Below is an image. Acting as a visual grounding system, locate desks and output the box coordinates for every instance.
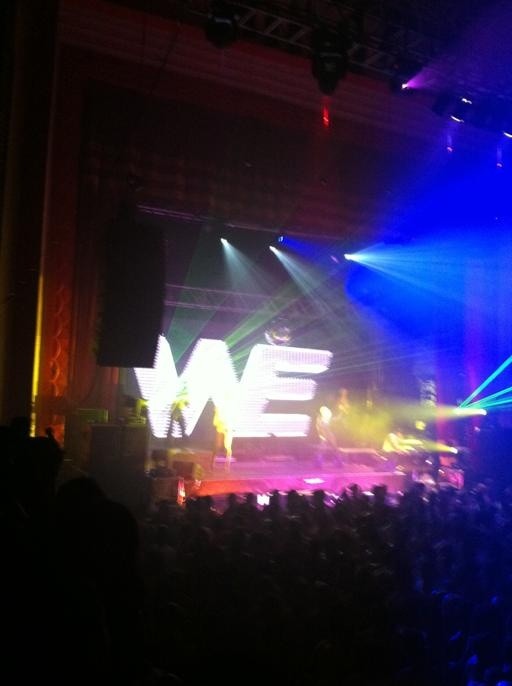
[63,417,108,459]
[89,423,148,471]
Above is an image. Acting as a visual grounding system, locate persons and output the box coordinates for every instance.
[211,396,234,474]
[337,387,354,417]
[0,414,512,686]
[166,386,190,438]
[315,405,346,470]
